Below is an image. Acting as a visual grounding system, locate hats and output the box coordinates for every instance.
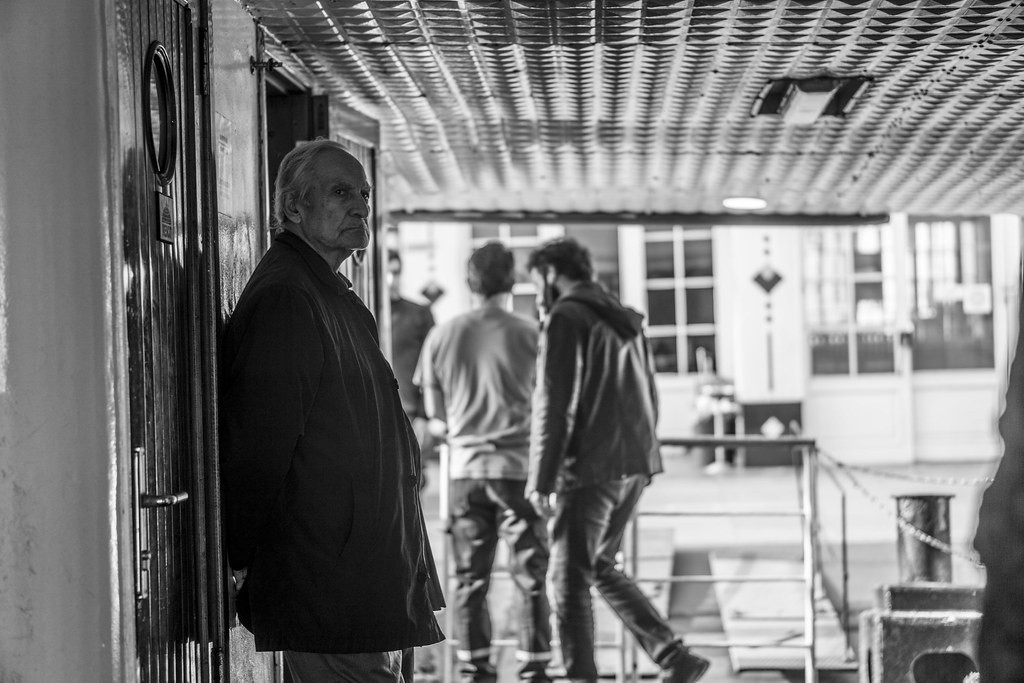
[468,243,514,294]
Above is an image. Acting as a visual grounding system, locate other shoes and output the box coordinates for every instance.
[659,642,707,683]
[516,661,552,683]
[457,662,497,683]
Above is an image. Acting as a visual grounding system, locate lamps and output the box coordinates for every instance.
[751,79,869,125]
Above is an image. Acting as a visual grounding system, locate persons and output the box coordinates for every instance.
[410,241,552,683]
[218,135,449,683]
[385,247,437,490]
[525,234,709,683]
